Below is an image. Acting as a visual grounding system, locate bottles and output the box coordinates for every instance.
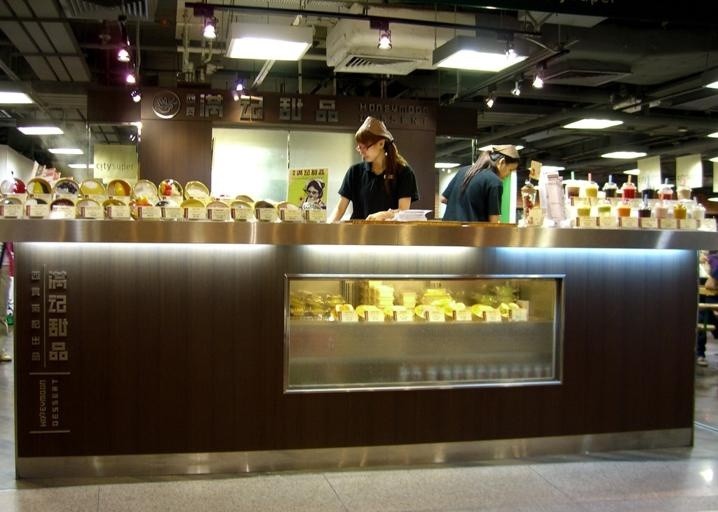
[546,171,566,223]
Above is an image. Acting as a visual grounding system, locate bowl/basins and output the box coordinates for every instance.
[27,177,53,220]
[207,195,327,223]
[157,179,185,220]
[184,181,210,219]
[106,178,131,220]
[52,179,80,221]
[131,180,162,220]
[79,179,106,220]
[0,176,26,218]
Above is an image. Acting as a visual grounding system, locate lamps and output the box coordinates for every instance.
[432,26,531,74]
[224,7,316,62]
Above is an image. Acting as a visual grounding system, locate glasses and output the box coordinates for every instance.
[355,140,380,152]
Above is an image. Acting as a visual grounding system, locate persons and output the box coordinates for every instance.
[332,115,421,222]
[298,177,328,209]
[439,142,521,224]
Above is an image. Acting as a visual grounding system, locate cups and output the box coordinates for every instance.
[563,171,707,233]
[520,184,537,220]
